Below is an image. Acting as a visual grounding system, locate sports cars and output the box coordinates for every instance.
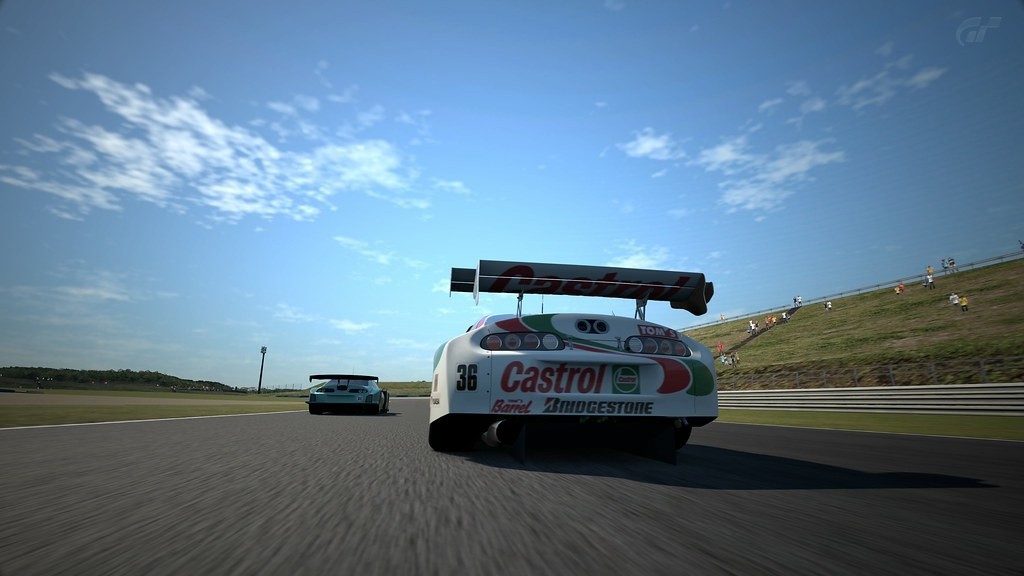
[428,259,720,458]
[305,374,390,416]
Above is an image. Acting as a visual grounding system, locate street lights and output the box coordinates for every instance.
[258,345,268,394]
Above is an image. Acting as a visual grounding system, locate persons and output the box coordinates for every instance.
[721,313,725,323]
[747,310,790,336]
[793,296,802,308]
[88,379,95,385]
[823,300,832,312]
[170,384,222,392]
[949,292,969,312]
[1020,242,1024,250]
[19,385,22,390]
[717,341,740,368]
[922,256,960,290]
[157,384,159,387]
[893,281,905,295]
[105,380,112,385]
[31,375,54,389]
